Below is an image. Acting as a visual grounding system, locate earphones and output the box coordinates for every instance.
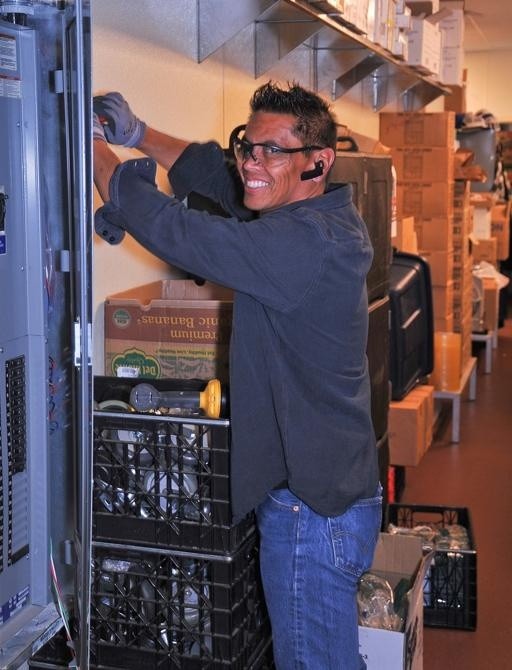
[300,161,325,180]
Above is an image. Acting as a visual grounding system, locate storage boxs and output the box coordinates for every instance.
[303,1,465,89]
[103,276,236,384]
[357,530,437,670]
[329,112,512,469]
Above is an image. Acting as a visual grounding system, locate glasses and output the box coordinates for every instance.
[227,124,325,166]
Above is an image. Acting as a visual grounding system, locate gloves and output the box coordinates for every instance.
[90,91,146,146]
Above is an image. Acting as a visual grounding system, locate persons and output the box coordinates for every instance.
[93,81,385,670]
